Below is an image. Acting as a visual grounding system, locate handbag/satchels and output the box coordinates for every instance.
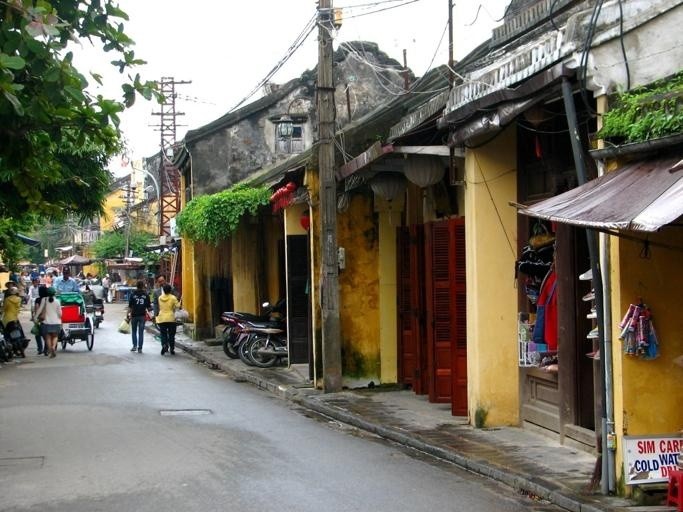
[118,317,132,335]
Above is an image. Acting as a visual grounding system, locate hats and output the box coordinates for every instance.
[5,280,18,288]
[9,287,19,295]
[63,267,71,274]
[47,287,56,295]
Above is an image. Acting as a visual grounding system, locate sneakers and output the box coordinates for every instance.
[161,345,175,355]
[130,346,143,354]
[38,348,56,358]
[578,267,600,362]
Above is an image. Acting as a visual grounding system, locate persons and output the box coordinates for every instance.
[137,273,146,285]
[153,274,175,352]
[147,274,154,288]
[126,281,153,354]
[156,284,182,355]
[1,265,111,359]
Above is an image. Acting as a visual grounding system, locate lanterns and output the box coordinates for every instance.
[403,154,445,217]
[271,181,297,212]
[371,174,408,225]
[300,215,310,232]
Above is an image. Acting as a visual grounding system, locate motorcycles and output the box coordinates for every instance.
[94,299,105,328]
[0,321,31,362]
[222,302,287,368]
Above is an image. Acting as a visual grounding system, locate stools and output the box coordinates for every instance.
[667,470,682,512]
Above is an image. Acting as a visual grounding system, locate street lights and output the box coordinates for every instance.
[131,166,160,237]
[272,97,342,392]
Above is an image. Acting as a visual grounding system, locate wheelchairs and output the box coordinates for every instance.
[59,292,94,350]
